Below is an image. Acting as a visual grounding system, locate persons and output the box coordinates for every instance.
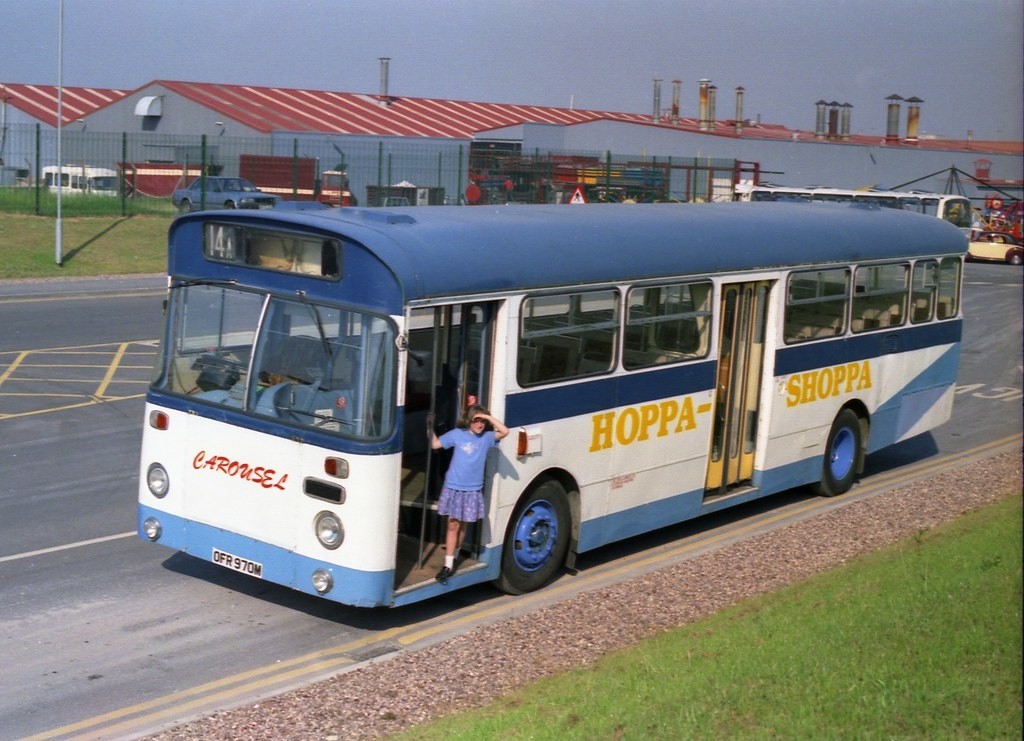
[426,404,509,580]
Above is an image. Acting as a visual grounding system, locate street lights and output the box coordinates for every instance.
[0,96,13,163]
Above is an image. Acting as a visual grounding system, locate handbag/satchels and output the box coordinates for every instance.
[195,367,240,391]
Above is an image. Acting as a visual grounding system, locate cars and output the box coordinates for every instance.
[963,231,1024,265]
[172,176,281,213]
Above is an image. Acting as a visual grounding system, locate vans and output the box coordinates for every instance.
[41,165,119,198]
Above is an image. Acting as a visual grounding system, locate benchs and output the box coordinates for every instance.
[445,281,953,383]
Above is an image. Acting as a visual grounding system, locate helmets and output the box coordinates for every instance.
[506,175,511,179]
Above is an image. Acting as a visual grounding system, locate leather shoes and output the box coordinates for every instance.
[435,557,460,580]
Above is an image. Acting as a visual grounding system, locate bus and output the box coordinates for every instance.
[135,202,972,609]
[735,184,974,247]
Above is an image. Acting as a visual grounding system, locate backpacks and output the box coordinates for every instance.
[549,347,570,379]
[274,378,354,437]
